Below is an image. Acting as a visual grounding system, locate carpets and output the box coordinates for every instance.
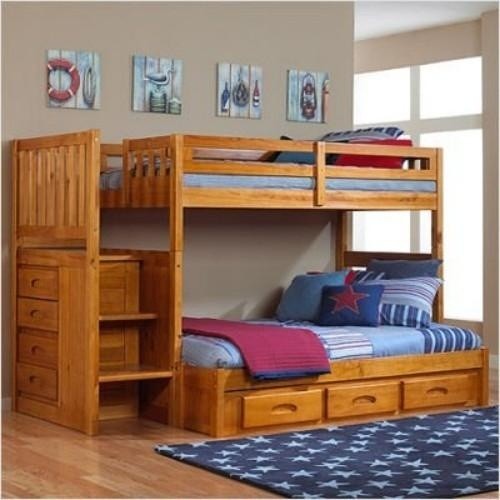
[155,404,499,498]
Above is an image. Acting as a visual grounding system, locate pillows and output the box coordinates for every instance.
[259,123,411,170]
[275,257,444,330]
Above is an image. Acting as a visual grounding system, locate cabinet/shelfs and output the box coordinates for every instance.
[14,256,141,422]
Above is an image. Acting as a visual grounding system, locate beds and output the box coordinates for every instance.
[100,131,491,438]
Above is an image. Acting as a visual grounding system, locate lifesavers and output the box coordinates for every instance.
[46,59,80,100]
[82,65,97,106]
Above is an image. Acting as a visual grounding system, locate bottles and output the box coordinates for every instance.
[220,79,232,113]
[252,79,260,109]
